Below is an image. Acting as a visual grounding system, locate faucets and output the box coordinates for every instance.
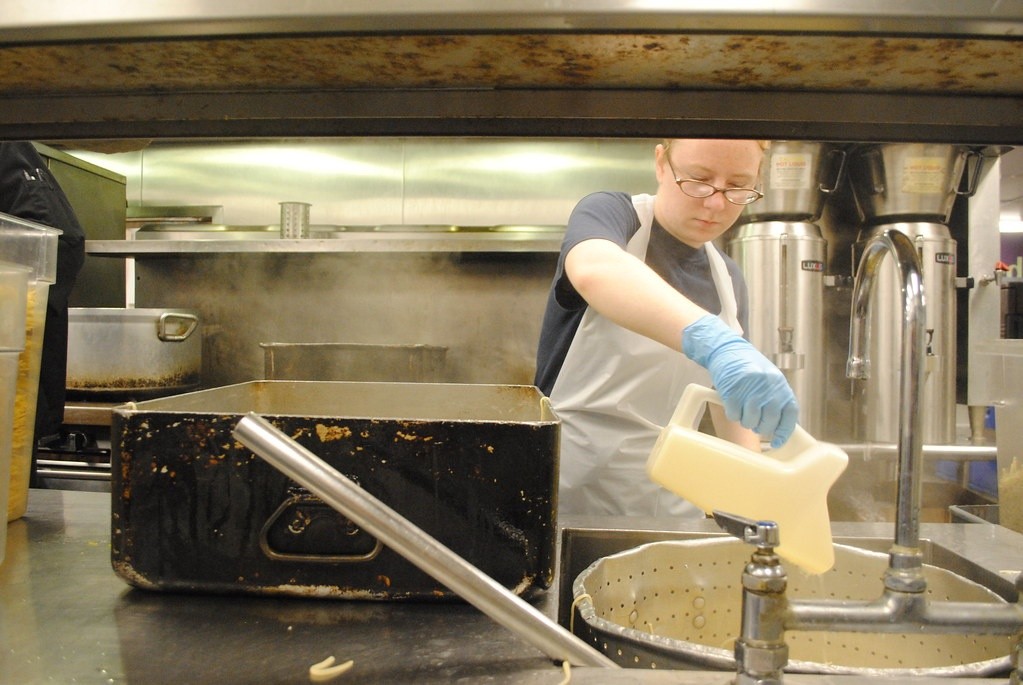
[844,225,935,636]
[981,262,1011,290]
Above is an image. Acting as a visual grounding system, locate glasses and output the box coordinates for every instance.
[664,146,765,206]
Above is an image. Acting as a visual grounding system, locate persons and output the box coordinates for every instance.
[532,135,798,536]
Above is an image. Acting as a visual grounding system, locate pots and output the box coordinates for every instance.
[259,339,452,385]
[67,306,202,393]
[109,377,565,603]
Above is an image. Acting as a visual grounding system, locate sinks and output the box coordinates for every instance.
[551,515,1023,685]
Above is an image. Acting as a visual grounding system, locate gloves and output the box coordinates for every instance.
[682,314,798,449]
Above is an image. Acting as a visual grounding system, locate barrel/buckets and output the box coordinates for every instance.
[718,218,830,443]
[844,221,958,449]
